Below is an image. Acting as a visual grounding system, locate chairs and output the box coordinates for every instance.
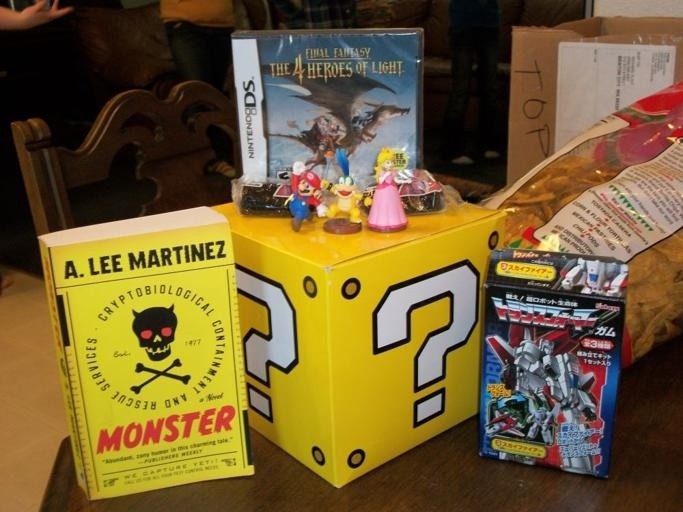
[10,79,243,238]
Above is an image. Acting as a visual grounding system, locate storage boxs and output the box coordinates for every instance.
[203,203,509,489]
[507,15,683,186]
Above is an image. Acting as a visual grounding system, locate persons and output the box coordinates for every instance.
[285,162,328,232]
[366,147,407,231]
[319,176,371,234]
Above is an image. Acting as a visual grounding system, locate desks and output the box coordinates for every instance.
[39,329,683,512]
